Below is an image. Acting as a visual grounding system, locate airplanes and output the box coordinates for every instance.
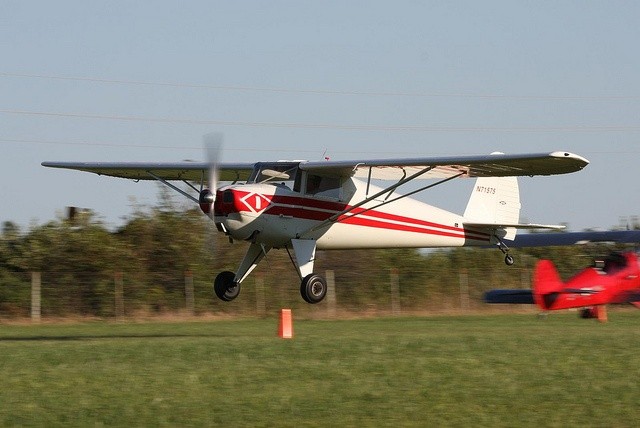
[41,151,590,306]
[481,229,640,323]
